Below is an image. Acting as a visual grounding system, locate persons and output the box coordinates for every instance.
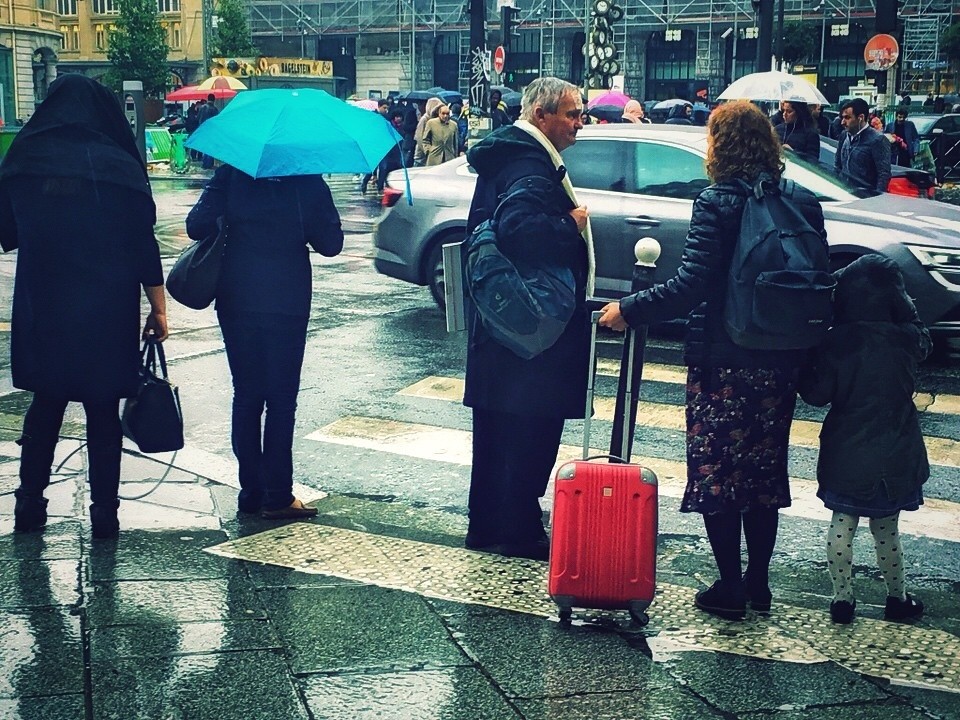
[463,76,597,562]
[801,253,933,623]
[580,92,936,166]
[884,109,919,167]
[361,94,467,193]
[835,98,891,192]
[0,74,169,541]
[491,90,513,132]
[600,100,831,618]
[186,163,344,519]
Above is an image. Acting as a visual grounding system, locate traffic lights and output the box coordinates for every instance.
[876,0,906,43]
[500,6,521,44]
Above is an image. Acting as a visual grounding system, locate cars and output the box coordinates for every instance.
[904,114,960,165]
[373,124,960,338]
[818,135,936,203]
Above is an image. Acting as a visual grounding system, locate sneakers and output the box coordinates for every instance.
[741,572,773,611]
[695,572,746,617]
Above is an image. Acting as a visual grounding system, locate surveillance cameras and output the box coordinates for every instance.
[721,28,733,38]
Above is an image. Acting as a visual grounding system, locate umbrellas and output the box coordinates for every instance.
[406,90,447,104]
[426,87,446,93]
[716,71,831,107]
[490,85,523,106]
[165,76,414,205]
[586,92,632,108]
[693,104,712,113]
[436,91,462,97]
[653,99,694,109]
[587,105,624,123]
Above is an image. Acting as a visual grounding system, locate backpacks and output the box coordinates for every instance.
[464,177,578,361]
[693,179,839,352]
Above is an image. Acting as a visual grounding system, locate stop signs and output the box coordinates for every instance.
[865,34,899,71]
[495,46,504,74]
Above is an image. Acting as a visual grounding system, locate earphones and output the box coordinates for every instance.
[859,118,862,122]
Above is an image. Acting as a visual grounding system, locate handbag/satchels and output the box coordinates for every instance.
[121,333,184,453]
[165,215,229,309]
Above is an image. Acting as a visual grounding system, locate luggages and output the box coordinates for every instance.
[548,309,658,627]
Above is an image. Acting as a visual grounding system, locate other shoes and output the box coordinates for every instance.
[465,533,551,560]
[378,190,385,196]
[885,592,924,619]
[90,521,120,539]
[13,517,47,533]
[351,175,375,183]
[239,498,318,520]
[830,599,856,624]
